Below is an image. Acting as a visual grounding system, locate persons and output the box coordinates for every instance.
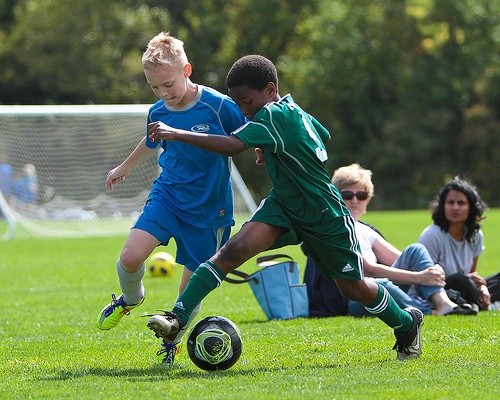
[0,152,39,220]
[418,182,500,310]
[147,55,424,360]
[96,32,265,365]
[303,165,480,316]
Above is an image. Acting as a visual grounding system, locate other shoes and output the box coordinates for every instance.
[444,303,477,316]
[488,301,500,310]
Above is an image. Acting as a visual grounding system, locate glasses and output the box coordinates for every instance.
[340,190,368,201]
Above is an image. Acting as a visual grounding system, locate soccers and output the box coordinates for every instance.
[187,316,242,373]
[148,252,175,278]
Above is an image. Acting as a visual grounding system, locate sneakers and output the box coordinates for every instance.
[394,307,424,360]
[159,340,183,368]
[96,289,146,330]
[147,314,179,339]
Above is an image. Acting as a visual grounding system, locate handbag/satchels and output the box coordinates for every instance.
[224,253,309,320]
